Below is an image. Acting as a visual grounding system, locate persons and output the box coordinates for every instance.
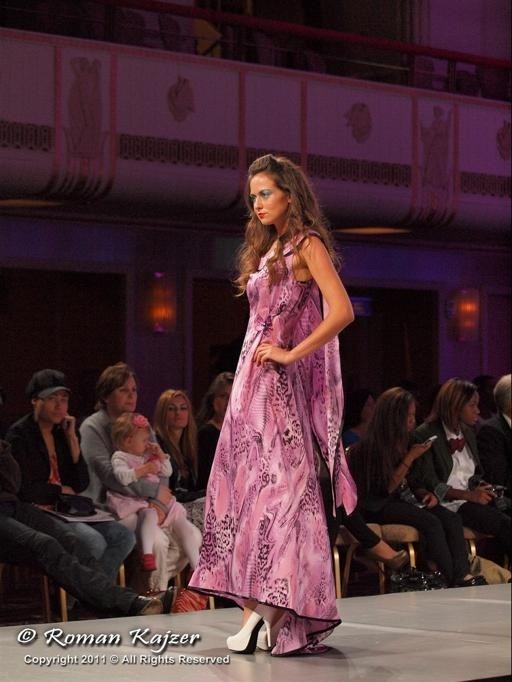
[0,360,511,616]
[188,152,361,657]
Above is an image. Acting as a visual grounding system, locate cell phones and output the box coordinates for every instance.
[423,435,438,445]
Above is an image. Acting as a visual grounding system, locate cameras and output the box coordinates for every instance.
[489,485,508,513]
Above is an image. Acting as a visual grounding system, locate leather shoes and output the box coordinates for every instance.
[140,586,179,615]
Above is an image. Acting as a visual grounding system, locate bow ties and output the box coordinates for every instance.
[448,439,468,453]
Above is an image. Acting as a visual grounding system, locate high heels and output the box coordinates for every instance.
[366,548,410,580]
[258,611,291,653]
[225,601,277,654]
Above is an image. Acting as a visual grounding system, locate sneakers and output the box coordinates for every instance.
[143,553,157,572]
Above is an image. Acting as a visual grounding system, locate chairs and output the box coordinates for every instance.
[0,419,512,617]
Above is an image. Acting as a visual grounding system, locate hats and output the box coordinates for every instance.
[29,368,73,401]
